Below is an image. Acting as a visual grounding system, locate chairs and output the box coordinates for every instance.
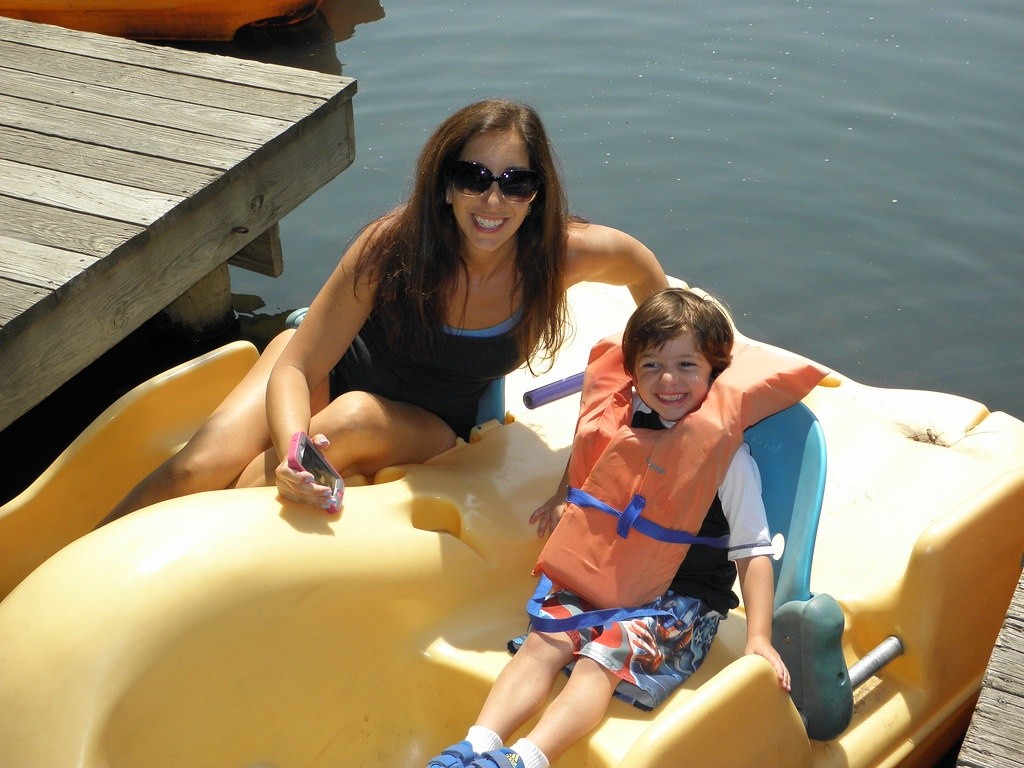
[742,401,827,613]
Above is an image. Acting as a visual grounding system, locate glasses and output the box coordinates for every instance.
[449,160,542,204]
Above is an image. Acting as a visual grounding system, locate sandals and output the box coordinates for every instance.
[464,746,525,768]
[425,740,481,768]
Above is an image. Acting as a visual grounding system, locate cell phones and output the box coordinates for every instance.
[288,431,345,513]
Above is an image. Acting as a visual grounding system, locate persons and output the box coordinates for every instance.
[424,289,792,768]
[89,101,672,532]
